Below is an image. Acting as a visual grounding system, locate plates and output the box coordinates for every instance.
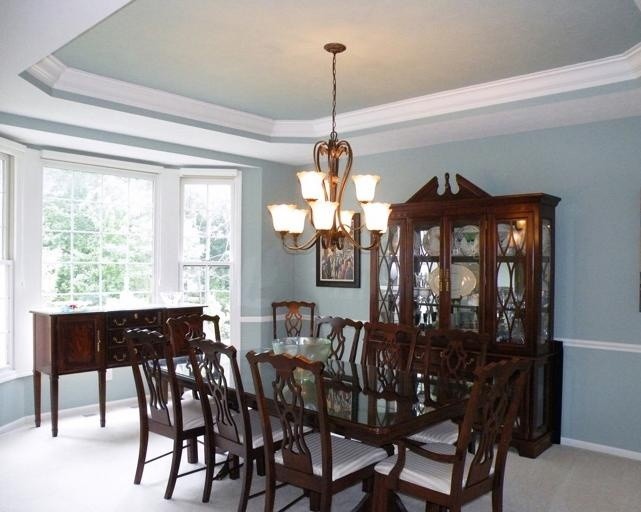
[455,225,479,256]
[429,264,476,299]
[423,227,454,256]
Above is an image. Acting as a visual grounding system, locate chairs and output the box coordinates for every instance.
[365,322,420,372]
[314,314,364,363]
[183,332,313,512]
[166,314,221,402]
[245,350,388,511]
[424,329,489,380]
[372,356,534,512]
[124,328,238,500]
[270,300,318,341]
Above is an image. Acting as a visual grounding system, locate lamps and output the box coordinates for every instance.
[266,44,391,251]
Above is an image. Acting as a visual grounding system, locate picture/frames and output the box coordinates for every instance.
[315,212,363,289]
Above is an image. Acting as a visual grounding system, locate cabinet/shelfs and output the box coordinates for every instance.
[364,173,563,460]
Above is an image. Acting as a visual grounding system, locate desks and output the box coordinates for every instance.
[148,350,481,512]
[28,299,206,436]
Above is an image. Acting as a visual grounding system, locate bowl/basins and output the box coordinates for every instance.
[272,336,331,381]
